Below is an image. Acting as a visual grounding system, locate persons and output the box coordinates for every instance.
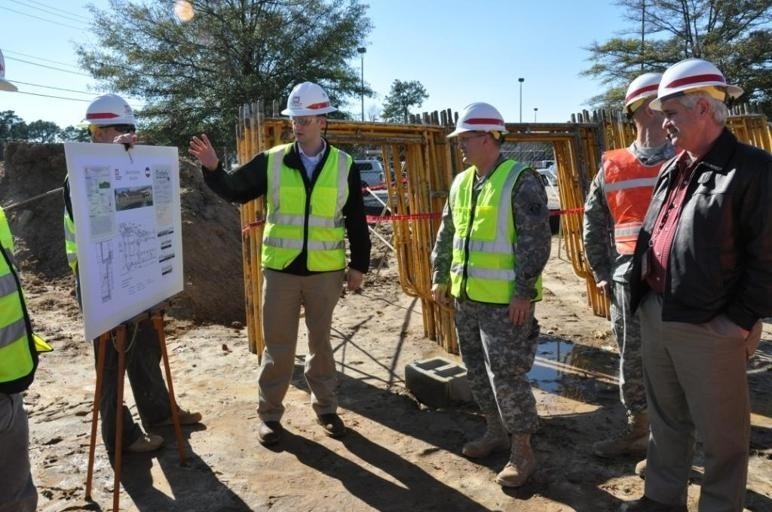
[187,76,372,448]
[580,65,697,479]
[625,56,772,512]
[424,99,550,494]
[62,96,203,454]
[0,49,56,512]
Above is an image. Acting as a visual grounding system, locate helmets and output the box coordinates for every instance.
[86,94,137,126]
[623,72,664,114]
[446,102,506,139]
[281,82,338,117]
[650,59,743,113]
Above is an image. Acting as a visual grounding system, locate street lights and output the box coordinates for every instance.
[356,47,367,122]
[534,108,538,122]
[517,77,524,123]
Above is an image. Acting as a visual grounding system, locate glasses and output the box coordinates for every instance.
[99,124,136,135]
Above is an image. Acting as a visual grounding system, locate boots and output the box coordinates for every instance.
[594,409,648,457]
[462,413,509,457]
[637,459,646,476]
[497,432,536,487]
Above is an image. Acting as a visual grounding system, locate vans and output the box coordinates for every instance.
[353,159,386,196]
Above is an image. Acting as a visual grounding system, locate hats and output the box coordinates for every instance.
[0,51,19,94]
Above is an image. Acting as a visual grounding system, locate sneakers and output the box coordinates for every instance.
[626,497,689,512]
[258,420,281,444]
[142,410,200,428]
[127,431,163,452]
[319,414,345,437]
